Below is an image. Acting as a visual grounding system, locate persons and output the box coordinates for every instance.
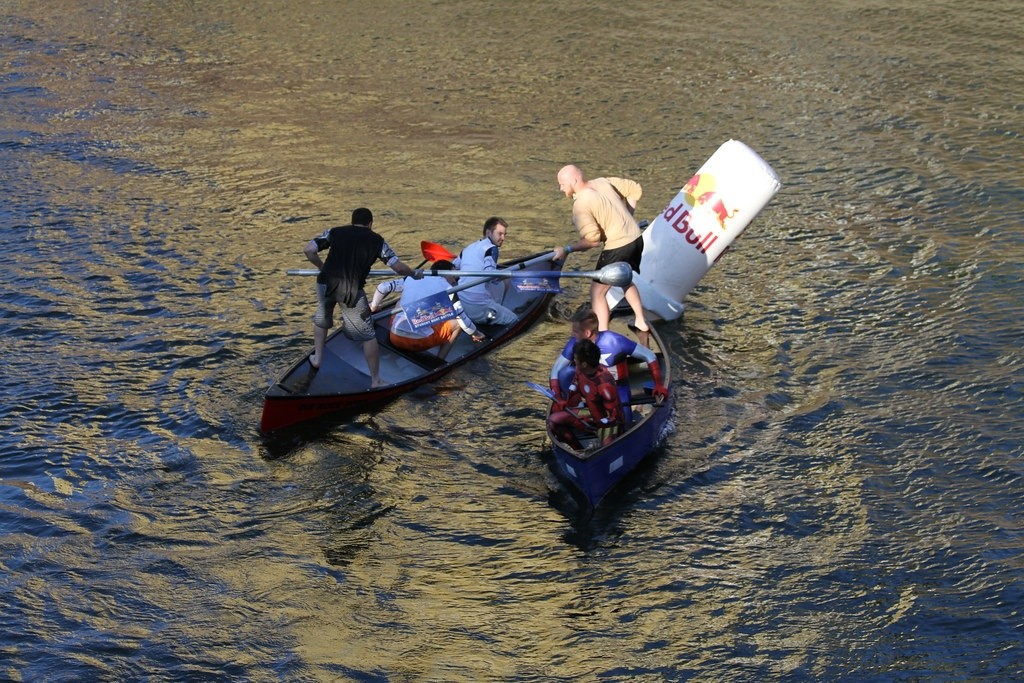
[303,208,424,388]
[548,338,624,447]
[369,259,485,361]
[549,309,668,428]
[551,164,649,332]
[450,216,518,325]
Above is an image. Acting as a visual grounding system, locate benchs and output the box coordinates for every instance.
[583,394,655,408]
[473,322,505,338]
[373,322,447,371]
[627,351,663,366]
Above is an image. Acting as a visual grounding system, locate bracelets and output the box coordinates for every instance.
[414,269,419,277]
[568,245,572,252]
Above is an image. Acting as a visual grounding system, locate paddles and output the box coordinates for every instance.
[383,258,427,299]
[525,381,597,430]
[421,241,508,269]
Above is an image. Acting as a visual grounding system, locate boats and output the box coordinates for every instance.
[547,303,674,514]
[262,246,567,430]
